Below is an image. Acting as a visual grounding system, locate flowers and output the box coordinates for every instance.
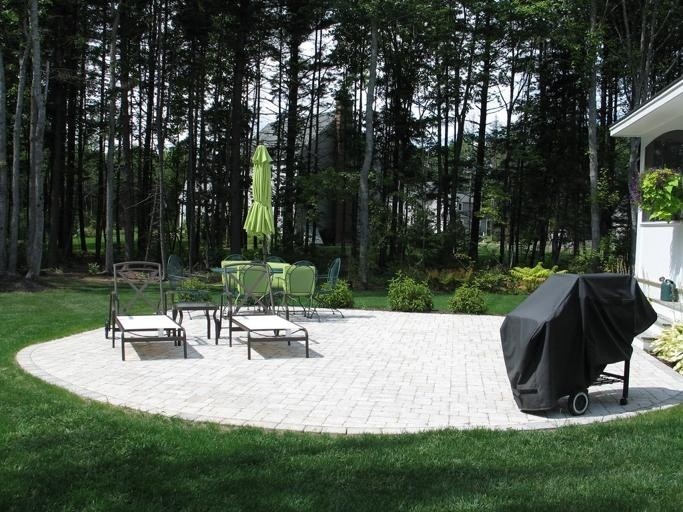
[627,166,683,223]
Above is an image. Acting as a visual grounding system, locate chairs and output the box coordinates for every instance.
[104,253,344,361]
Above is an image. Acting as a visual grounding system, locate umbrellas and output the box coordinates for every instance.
[240,147,275,265]
